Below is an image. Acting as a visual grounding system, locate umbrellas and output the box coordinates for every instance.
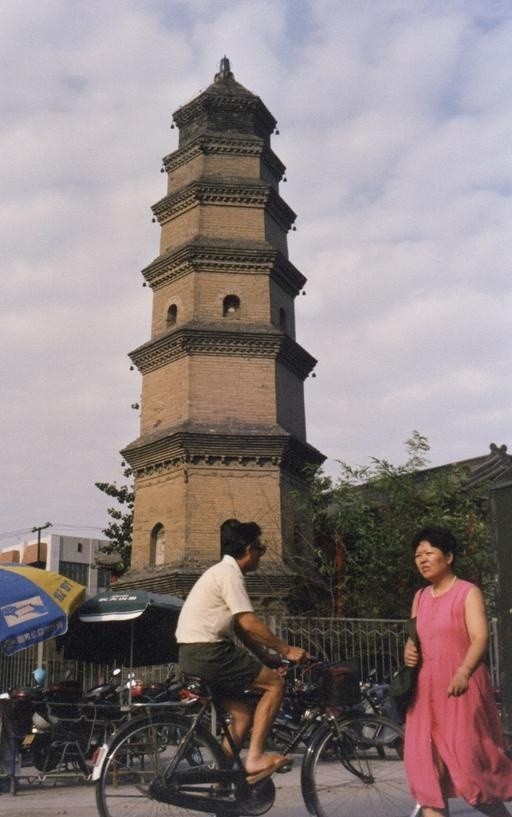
[56,591,185,768]
[0,563,85,673]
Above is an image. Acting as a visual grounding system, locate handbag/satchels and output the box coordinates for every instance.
[386,617,422,705]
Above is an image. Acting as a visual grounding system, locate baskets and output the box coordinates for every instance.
[301,657,362,707]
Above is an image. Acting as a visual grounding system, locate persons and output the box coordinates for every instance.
[401,525,512,817]
[173,522,311,798]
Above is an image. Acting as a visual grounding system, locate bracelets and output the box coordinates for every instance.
[459,664,473,671]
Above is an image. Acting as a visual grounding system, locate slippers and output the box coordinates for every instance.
[246,750,292,785]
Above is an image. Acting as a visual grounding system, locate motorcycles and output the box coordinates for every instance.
[1,670,203,772]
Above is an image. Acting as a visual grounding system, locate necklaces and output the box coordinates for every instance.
[430,573,457,599]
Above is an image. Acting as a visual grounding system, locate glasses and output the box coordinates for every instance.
[258,544,266,556]
